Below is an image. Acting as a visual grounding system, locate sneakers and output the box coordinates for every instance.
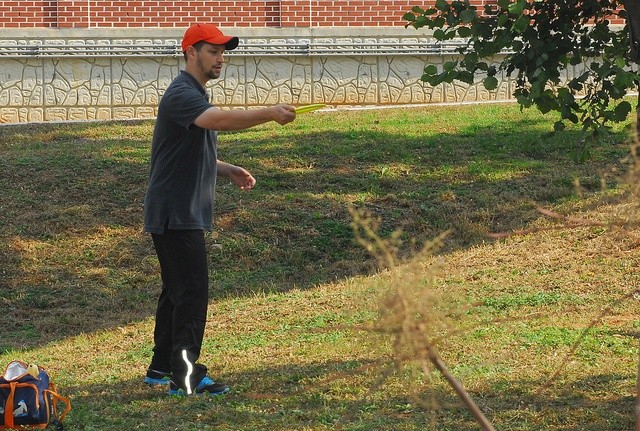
[169,374,230,395]
[144,366,171,385]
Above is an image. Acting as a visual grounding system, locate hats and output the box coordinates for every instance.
[182,24,239,53]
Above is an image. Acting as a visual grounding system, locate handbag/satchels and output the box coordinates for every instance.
[0,361,53,430]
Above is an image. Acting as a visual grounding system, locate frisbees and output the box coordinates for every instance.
[295,103,326,114]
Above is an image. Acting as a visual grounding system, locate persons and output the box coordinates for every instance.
[143,23,296,398]
